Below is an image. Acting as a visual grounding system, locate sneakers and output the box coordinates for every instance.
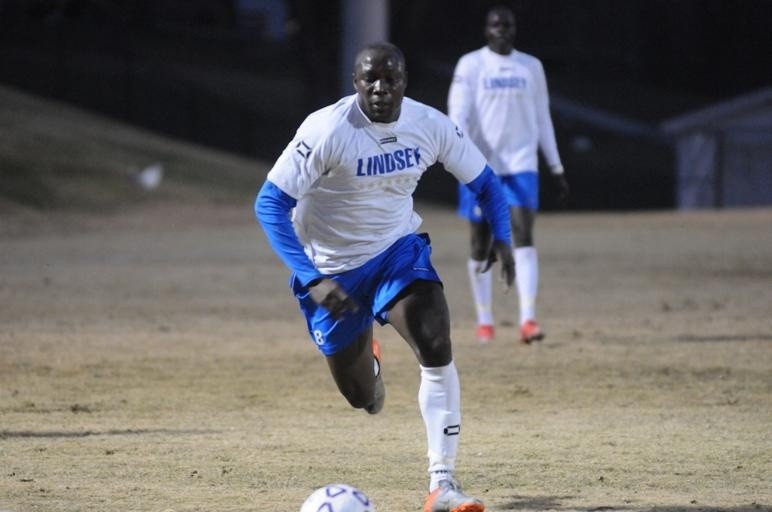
[365,339,385,415]
[519,321,546,343]
[478,325,496,343]
[423,481,484,512]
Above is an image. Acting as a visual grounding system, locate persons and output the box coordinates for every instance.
[253,40,517,512]
[444,7,570,345]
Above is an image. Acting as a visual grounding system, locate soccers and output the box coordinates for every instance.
[300,484,373,511]
